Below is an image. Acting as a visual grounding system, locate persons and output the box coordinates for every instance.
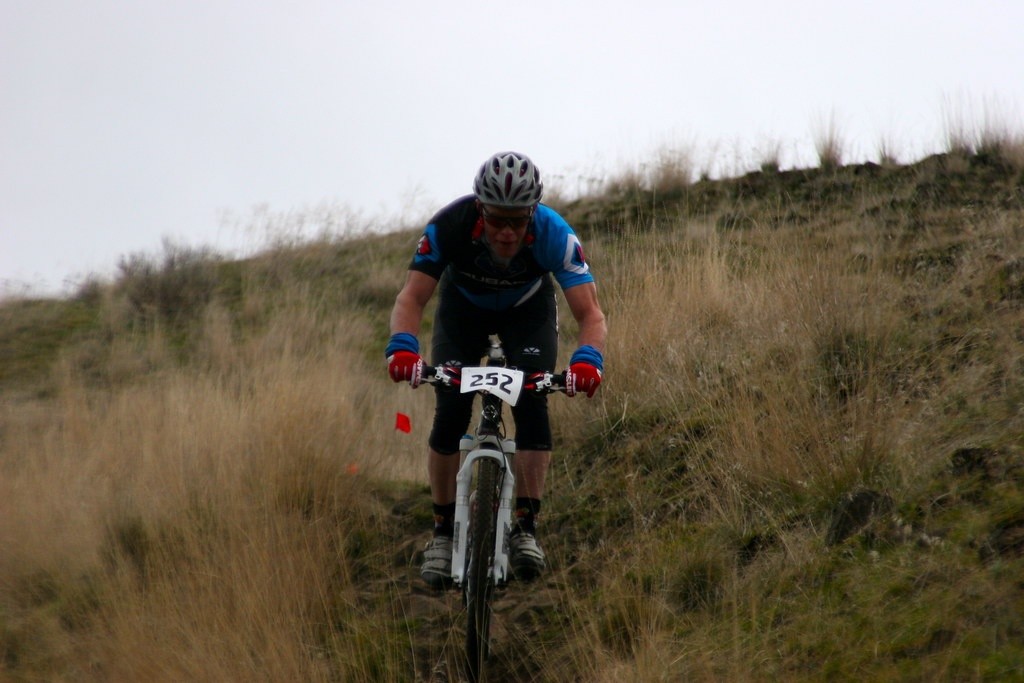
[384,151,612,594]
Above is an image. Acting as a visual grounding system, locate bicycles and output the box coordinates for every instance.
[409,341,582,683]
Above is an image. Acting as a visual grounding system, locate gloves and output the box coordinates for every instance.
[559,362,602,398]
[386,350,428,389]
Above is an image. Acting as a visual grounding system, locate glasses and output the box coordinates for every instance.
[480,205,535,228]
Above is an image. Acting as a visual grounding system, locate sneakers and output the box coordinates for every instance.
[510,533,546,577]
[420,537,452,583]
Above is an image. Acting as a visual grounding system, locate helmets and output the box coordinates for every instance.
[472,150,543,206]
